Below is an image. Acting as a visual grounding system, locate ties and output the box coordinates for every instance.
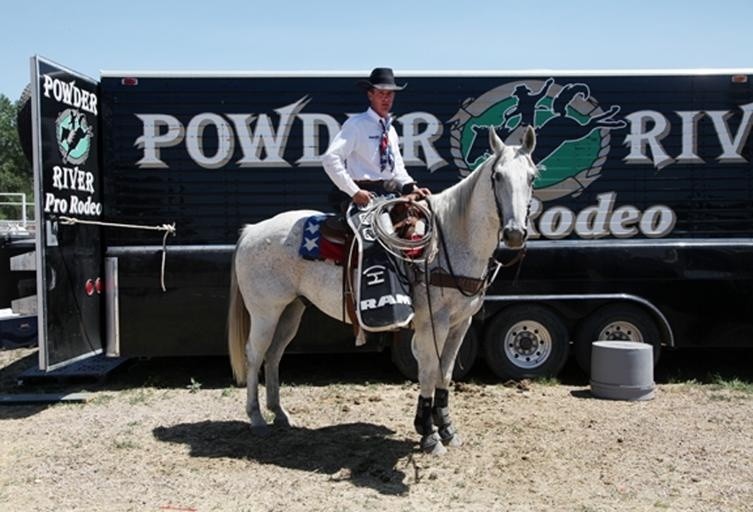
[380,121,396,174]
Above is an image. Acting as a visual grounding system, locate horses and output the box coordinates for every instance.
[227,124,541,454]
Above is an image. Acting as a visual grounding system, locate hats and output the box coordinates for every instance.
[358,67,408,92]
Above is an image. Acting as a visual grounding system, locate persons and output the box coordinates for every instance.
[321,67,433,217]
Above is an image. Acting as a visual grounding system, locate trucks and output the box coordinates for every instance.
[16,50,752,380]
